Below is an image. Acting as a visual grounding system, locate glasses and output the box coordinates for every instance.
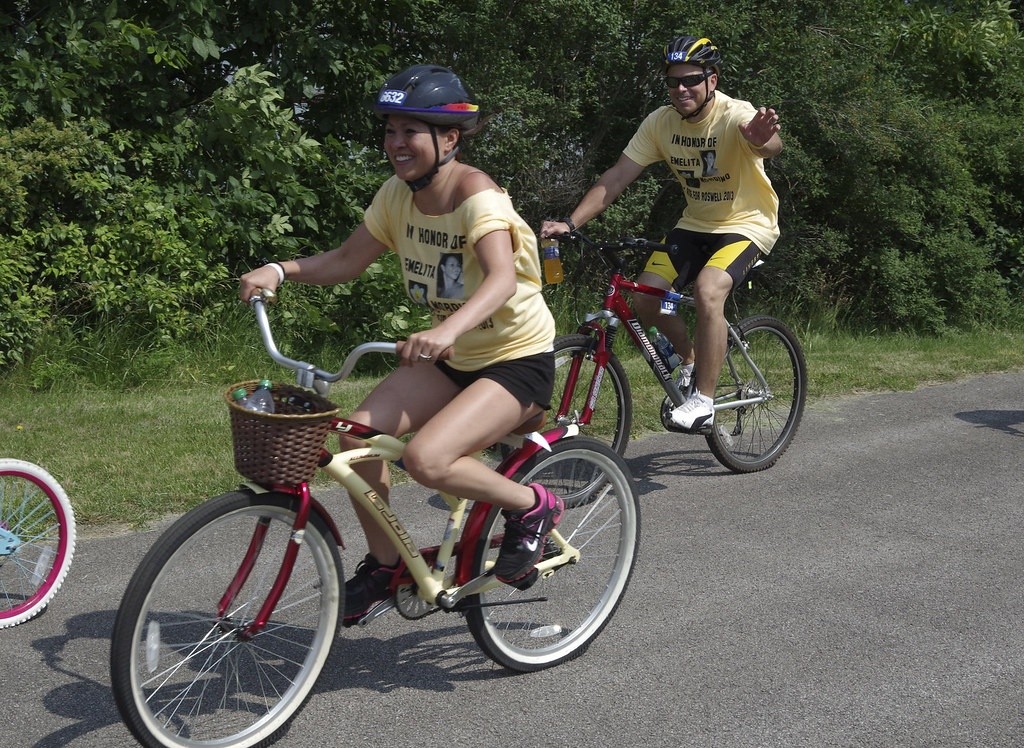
[666,71,715,88]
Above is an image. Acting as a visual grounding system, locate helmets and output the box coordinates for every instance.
[661,36,721,76]
[374,64,480,131]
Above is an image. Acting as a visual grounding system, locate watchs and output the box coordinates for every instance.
[559,216,575,232]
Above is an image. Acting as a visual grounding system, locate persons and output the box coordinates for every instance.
[541,37,785,430]
[242,65,565,622]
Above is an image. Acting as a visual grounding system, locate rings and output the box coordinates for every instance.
[419,354,431,361]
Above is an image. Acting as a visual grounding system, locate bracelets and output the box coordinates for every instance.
[264,261,285,286]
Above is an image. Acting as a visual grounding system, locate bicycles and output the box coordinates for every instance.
[2,458,78,632]
[107,288,646,748]
[500,230,809,511]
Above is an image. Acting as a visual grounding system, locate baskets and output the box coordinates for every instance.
[223,378,341,484]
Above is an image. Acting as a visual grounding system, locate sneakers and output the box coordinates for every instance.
[663,387,716,432]
[340,552,400,619]
[676,369,695,388]
[494,482,563,583]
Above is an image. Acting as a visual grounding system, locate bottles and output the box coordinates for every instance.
[246,379,275,414]
[232,387,251,408]
[541,238,564,284]
[649,326,680,368]
